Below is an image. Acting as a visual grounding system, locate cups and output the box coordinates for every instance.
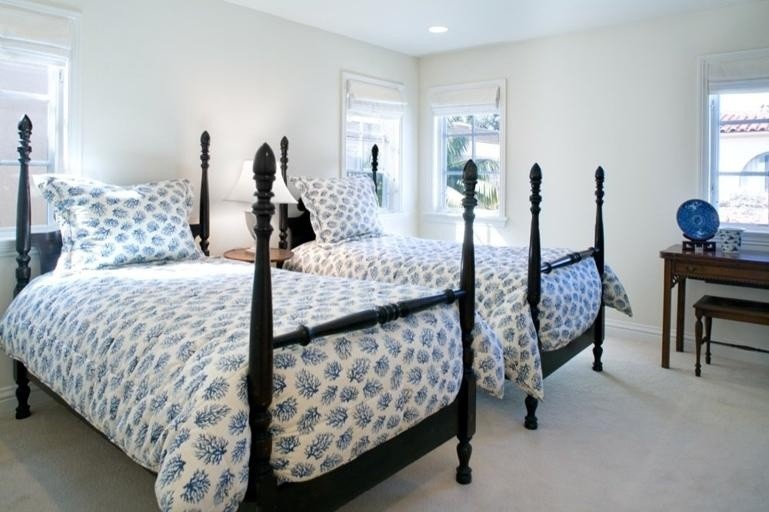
[718,227,744,256]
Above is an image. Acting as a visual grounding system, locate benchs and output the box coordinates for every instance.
[691,293,768,374]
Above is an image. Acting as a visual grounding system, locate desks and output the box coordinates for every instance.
[656,238,768,372]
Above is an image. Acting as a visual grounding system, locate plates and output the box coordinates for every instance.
[675,199,721,242]
